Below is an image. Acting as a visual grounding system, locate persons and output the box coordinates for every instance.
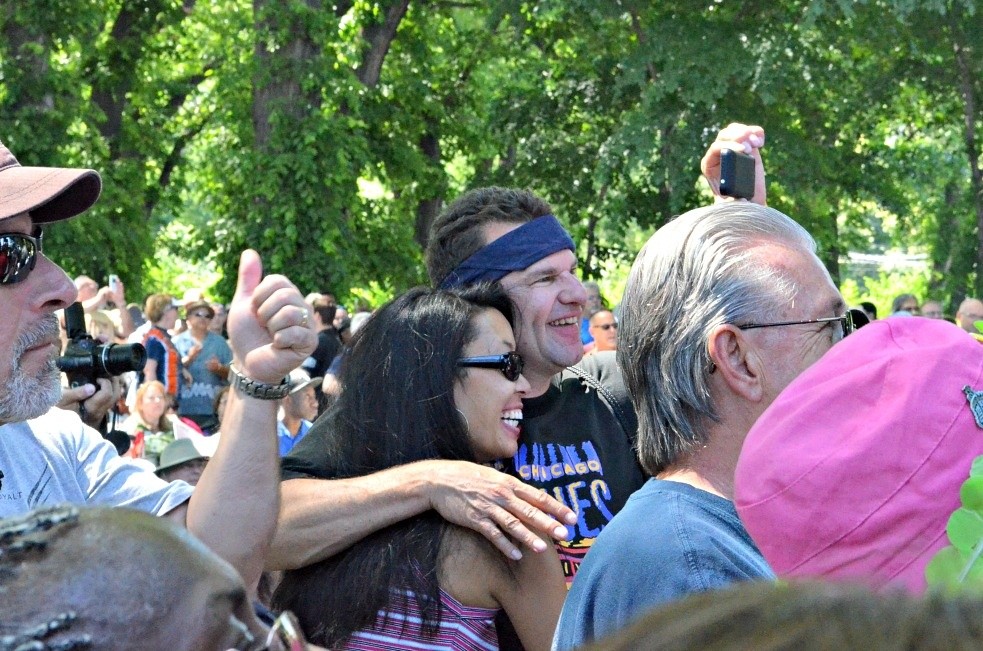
[0,507,329,651]
[0,145,320,582]
[554,203,853,651]
[261,123,765,651]
[46,274,983,454]
[560,581,983,651]
[733,315,983,581]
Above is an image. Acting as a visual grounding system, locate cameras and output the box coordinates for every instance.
[58,303,147,401]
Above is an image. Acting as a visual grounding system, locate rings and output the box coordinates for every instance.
[300,305,308,326]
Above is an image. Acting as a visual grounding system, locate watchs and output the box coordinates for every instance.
[227,360,292,401]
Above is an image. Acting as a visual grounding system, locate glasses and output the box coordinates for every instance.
[707,310,856,376]
[263,610,308,651]
[904,306,920,312]
[456,351,528,384]
[0,225,46,287]
[191,311,212,318]
[590,320,619,332]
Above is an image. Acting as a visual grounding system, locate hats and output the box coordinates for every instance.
[731,309,983,602]
[185,302,215,317]
[285,366,325,394]
[153,438,211,479]
[0,139,103,226]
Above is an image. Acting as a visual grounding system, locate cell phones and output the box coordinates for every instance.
[719,150,755,201]
